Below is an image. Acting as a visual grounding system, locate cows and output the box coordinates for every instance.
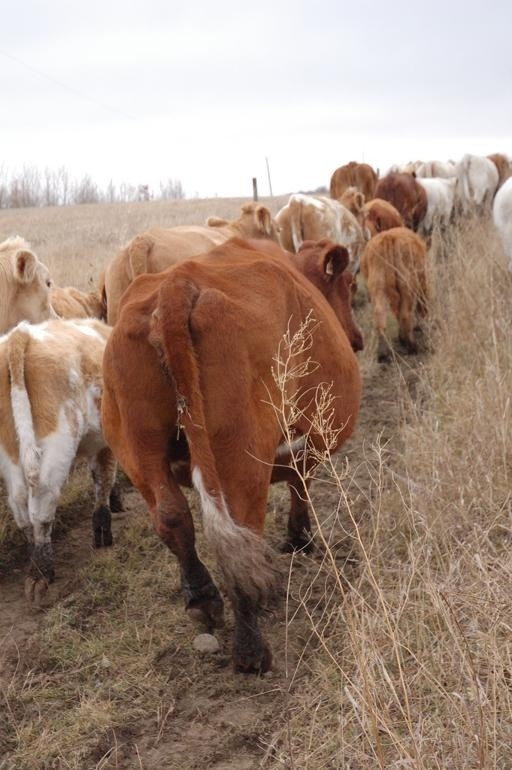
[274,150,512,364]
[104,201,284,324]
[0,236,60,339]
[0,316,127,602]
[51,284,101,320]
[100,236,364,674]
